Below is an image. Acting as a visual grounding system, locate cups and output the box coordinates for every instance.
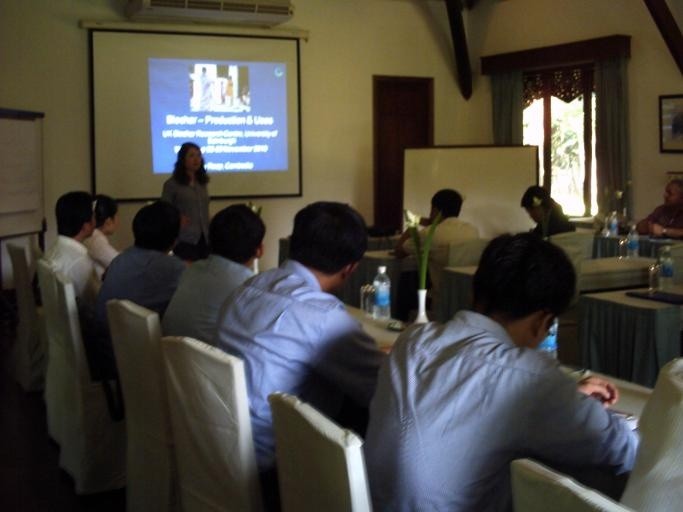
[601,217,609,238]
[648,264,661,294]
[360,285,374,321]
[617,238,630,264]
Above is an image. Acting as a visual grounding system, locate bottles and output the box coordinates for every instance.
[626,225,639,260]
[610,211,618,238]
[373,266,390,322]
[659,246,674,292]
[537,317,558,360]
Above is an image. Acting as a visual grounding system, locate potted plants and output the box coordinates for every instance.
[403,207,441,325]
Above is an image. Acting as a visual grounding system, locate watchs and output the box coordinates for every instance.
[661,226,667,238]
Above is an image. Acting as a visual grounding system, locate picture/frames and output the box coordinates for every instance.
[658,94,683,155]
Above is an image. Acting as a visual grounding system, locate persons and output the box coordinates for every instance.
[617,328,682,511]
[81,194,122,308]
[364,232,642,511]
[520,186,576,239]
[213,202,380,511]
[38,190,106,364]
[635,179,682,241]
[224,75,233,111]
[199,67,214,109]
[158,142,211,263]
[394,189,480,322]
[159,203,267,348]
[91,198,185,377]
